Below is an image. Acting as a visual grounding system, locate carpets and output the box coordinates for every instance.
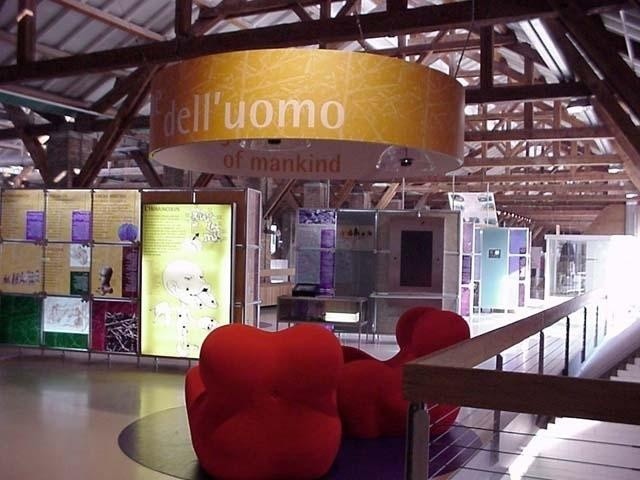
[117,403,485,479]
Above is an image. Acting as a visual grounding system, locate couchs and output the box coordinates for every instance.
[333,306,471,440]
[187,324,342,480]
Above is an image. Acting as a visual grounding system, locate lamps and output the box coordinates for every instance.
[146,1,467,185]
